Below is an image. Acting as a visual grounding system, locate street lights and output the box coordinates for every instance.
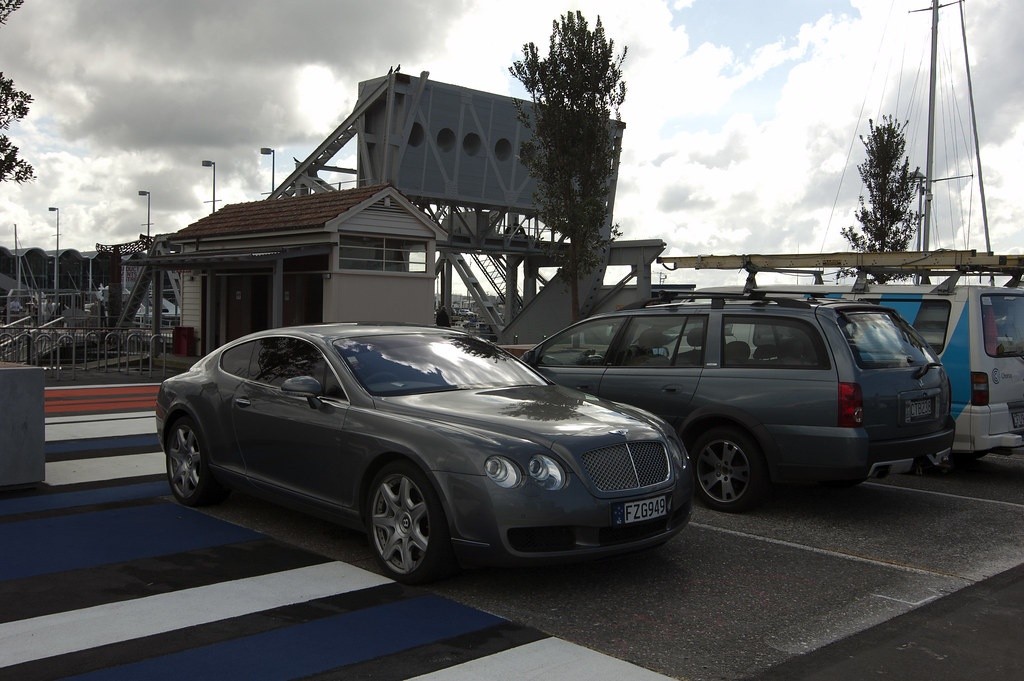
[48,206,60,316]
[261,147,275,194]
[83,255,92,304]
[138,190,152,333]
[201,160,216,214]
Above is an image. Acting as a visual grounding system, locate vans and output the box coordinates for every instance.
[681,284,1024,472]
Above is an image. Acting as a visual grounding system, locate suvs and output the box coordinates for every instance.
[518,290,958,513]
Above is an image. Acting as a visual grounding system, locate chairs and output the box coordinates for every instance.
[726,341,782,361]
[676,328,706,365]
[638,329,670,366]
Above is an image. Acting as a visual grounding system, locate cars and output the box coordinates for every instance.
[157,322,694,586]
